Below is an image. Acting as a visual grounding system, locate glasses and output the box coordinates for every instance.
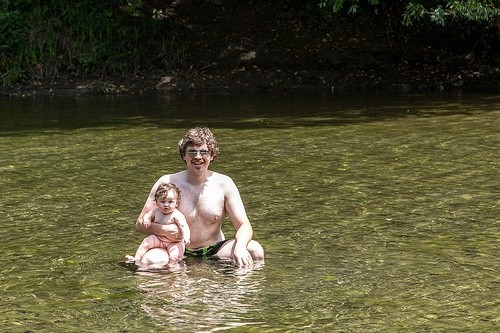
[186,150,210,157]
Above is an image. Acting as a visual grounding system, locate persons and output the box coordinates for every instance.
[125,182,190,273]
[135,126,268,273]
